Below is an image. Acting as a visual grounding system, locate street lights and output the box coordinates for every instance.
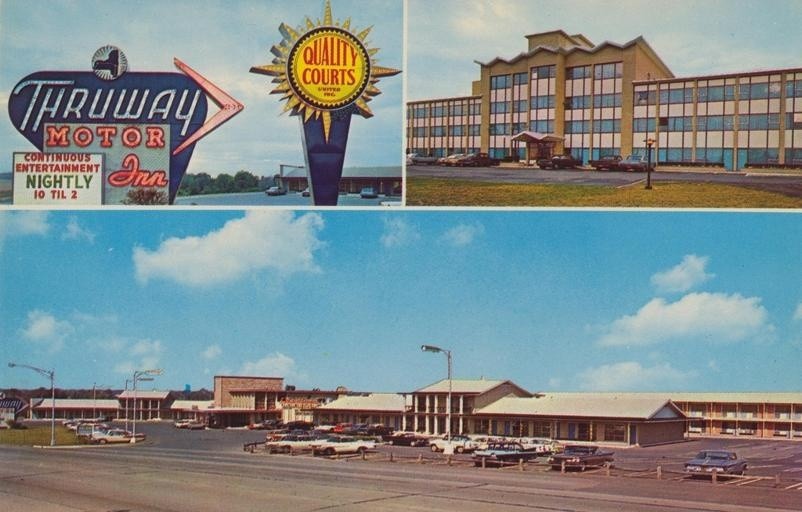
[421,345,451,465]
[124,379,154,432]
[131,367,164,443]
[9,362,56,446]
[642,138,656,189]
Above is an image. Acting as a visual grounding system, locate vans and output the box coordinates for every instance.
[360,187,377,198]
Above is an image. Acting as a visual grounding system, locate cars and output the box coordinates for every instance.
[617,155,656,172]
[265,186,287,196]
[471,440,538,467]
[537,154,583,170]
[547,444,614,472]
[406,152,500,168]
[587,154,623,171]
[682,449,749,481]
[302,187,310,198]
